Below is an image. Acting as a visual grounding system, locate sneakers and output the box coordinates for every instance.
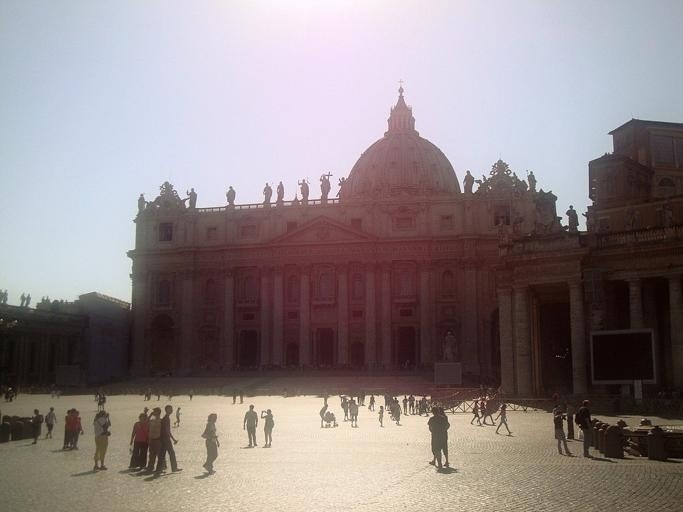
[428,459,451,470]
[248,441,272,448]
[62,445,80,451]
[92,464,107,474]
[495,431,513,435]
[46,433,52,439]
[470,421,496,427]
[126,462,217,477]
[556,450,594,459]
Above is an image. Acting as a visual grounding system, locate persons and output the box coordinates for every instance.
[189,389,193,400]
[226,186,235,204]
[233,390,244,405]
[138,194,147,214]
[244,404,274,448]
[263,183,272,204]
[463,170,474,193]
[128,405,184,472]
[320,175,330,204]
[94,387,107,412]
[298,179,309,199]
[528,171,537,191]
[187,188,197,208]
[338,177,345,189]
[199,414,221,472]
[29,407,112,472]
[552,392,592,458]
[143,386,173,401]
[426,406,451,468]
[566,206,579,232]
[318,385,514,436]
[4,381,62,402]
[276,181,284,201]
